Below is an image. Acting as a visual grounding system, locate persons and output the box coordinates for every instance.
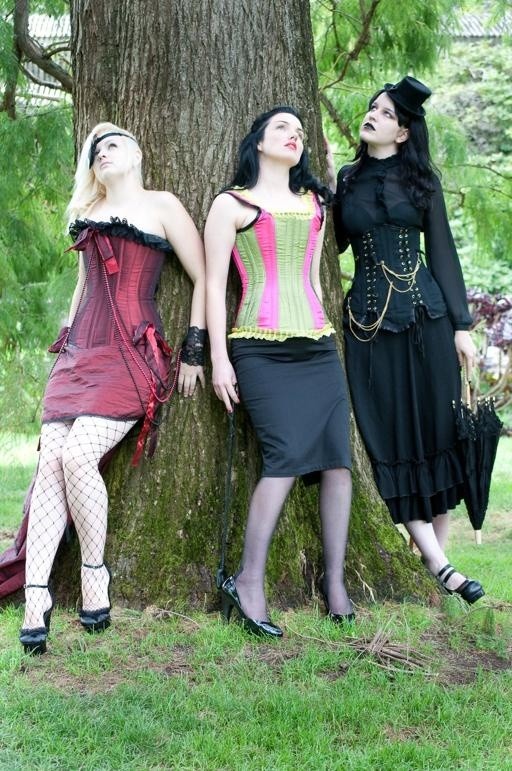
[203,106,359,637]
[19,122,206,655]
[323,77,486,605]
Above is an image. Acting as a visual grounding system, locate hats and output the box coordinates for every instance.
[384,76,432,116]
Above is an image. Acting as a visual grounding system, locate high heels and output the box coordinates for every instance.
[79,564,111,633]
[221,576,282,637]
[316,571,355,624]
[19,585,54,654]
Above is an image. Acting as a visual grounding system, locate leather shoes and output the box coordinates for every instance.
[434,564,484,604]
